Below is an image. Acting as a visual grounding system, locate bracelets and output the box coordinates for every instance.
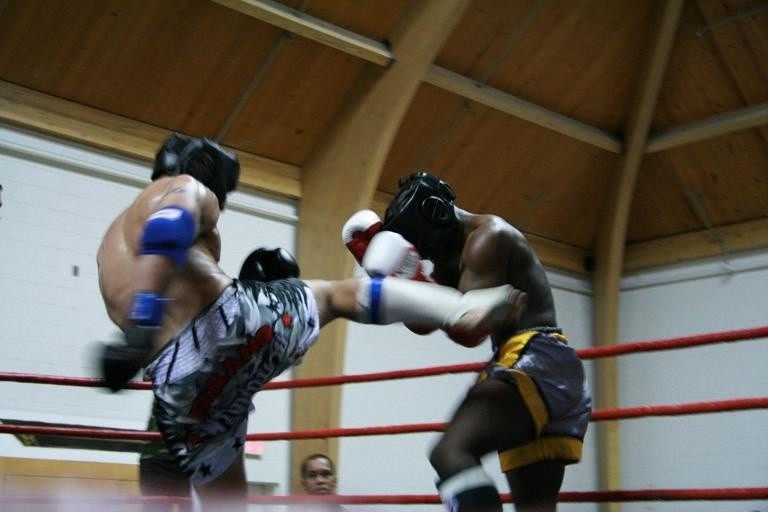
[129,289,165,327]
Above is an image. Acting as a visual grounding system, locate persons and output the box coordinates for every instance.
[342,172,589,512]
[95,131,527,510]
[138,406,193,511]
[299,453,336,495]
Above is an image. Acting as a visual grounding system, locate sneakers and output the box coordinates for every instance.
[444,284,527,347]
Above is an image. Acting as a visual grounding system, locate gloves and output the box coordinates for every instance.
[239,248,300,282]
[104,327,153,392]
[342,210,437,283]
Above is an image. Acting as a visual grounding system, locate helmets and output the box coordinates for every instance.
[151,133,240,209]
[384,172,463,259]
